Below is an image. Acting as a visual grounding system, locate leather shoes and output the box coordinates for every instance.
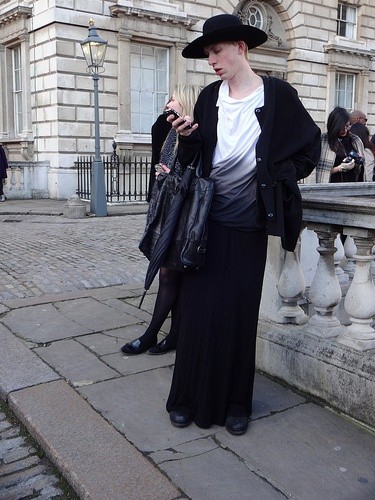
[120,335,158,356]
[146,337,176,355]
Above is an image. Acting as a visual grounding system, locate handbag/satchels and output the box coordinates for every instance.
[136,157,214,273]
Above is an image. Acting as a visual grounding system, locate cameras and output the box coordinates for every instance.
[348,150,366,165]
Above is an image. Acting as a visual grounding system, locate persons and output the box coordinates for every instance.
[0,145,8,201]
[165,15,321,435]
[316,107,375,246]
[121,84,205,356]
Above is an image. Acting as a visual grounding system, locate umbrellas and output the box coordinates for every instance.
[137,136,203,310]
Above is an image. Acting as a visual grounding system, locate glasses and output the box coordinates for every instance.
[342,124,353,130]
[361,117,368,121]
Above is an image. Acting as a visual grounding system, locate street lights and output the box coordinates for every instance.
[79,17,110,215]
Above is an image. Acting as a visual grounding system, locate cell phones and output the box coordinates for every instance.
[164,108,192,130]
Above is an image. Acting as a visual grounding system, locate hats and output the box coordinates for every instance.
[181,14,269,59]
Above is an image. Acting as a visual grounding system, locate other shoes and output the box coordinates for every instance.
[1,193,6,202]
[169,411,192,427]
[224,415,248,435]
